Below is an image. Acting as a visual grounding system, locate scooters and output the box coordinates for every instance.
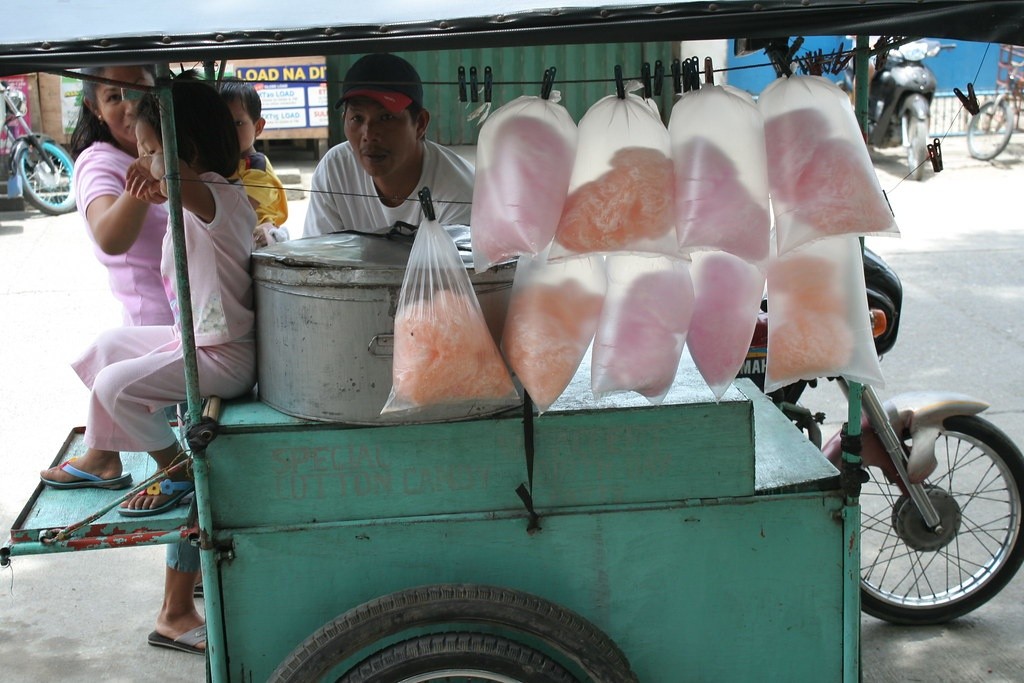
[0,0,1024,683]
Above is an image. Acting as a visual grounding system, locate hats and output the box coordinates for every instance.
[335,54,424,115]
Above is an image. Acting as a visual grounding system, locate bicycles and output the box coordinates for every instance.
[968,44,1024,162]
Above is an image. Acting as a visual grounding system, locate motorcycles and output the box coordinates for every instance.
[836,43,955,180]
[0,82,78,216]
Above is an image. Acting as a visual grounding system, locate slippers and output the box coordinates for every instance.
[148,622,206,655]
[116,465,195,517]
[40,456,132,491]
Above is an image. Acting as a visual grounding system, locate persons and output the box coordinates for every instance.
[69,65,206,655]
[215,76,288,228]
[40,68,257,518]
[301,54,477,238]
